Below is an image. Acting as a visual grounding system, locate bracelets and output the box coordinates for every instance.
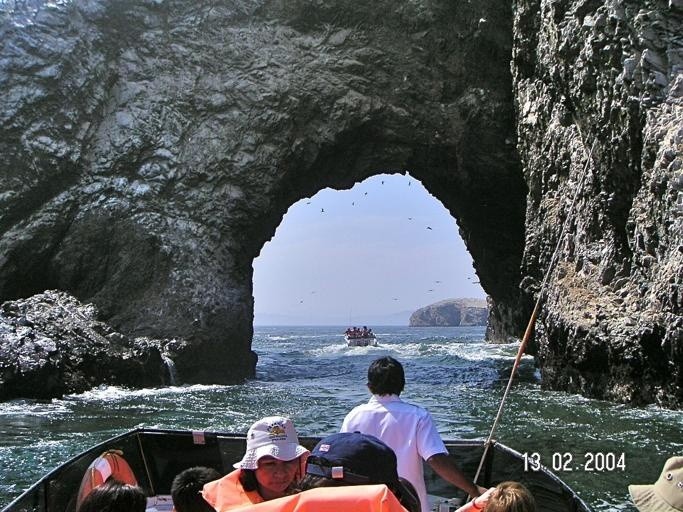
[472,497,482,511]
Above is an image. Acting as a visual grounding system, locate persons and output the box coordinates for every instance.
[204,415,311,512]
[398,476,423,511]
[451,481,537,511]
[628,454,682,512]
[79,479,147,512]
[296,431,416,512]
[170,466,222,512]
[345,325,374,338]
[340,356,480,512]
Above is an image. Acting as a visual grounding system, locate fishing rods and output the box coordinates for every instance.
[468,138,598,497]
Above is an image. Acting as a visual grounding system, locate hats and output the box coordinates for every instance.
[303,429,420,505]
[231,414,310,480]
[625,454,683,511]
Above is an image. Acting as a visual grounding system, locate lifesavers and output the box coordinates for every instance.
[79,452,138,505]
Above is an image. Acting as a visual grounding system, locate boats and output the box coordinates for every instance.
[343,327,378,347]
[0,426,592,511]
